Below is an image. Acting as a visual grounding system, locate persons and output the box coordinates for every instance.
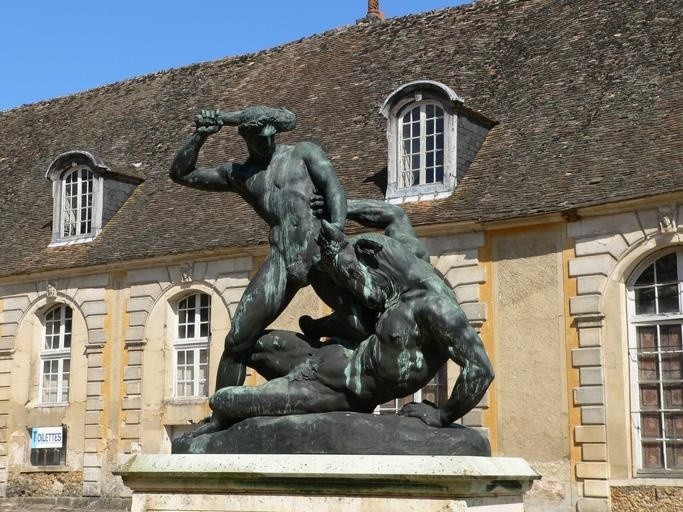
[207,188,495,428]
[170,109,346,439]
